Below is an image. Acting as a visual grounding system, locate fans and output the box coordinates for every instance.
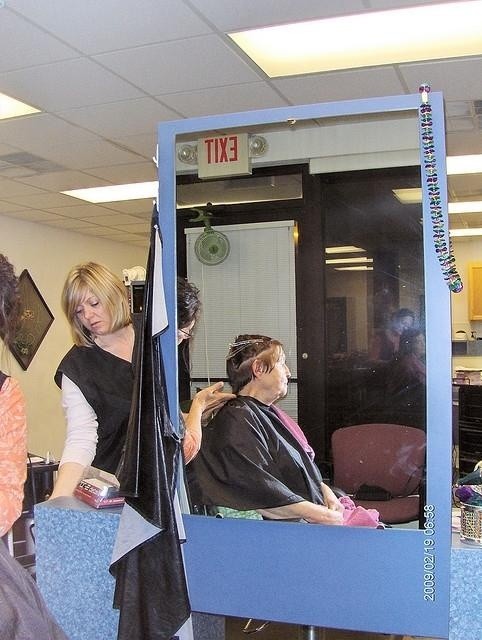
[189,205,231,267]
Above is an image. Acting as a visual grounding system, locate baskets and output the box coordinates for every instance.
[453,484,481,544]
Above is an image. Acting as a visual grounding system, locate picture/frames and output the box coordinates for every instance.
[1,268,56,370]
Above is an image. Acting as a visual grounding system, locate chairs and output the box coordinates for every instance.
[178,398,210,516]
[324,424,427,529]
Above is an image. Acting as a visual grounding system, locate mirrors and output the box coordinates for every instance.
[156,89,455,640]
[318,164,423,464]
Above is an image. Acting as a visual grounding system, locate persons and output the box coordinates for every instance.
[210,334,383,528]
[380,308,415,361]
[0,253,29,541]
[177,277,236,517]
[398,327,425,383]
[49,262,141,501]
